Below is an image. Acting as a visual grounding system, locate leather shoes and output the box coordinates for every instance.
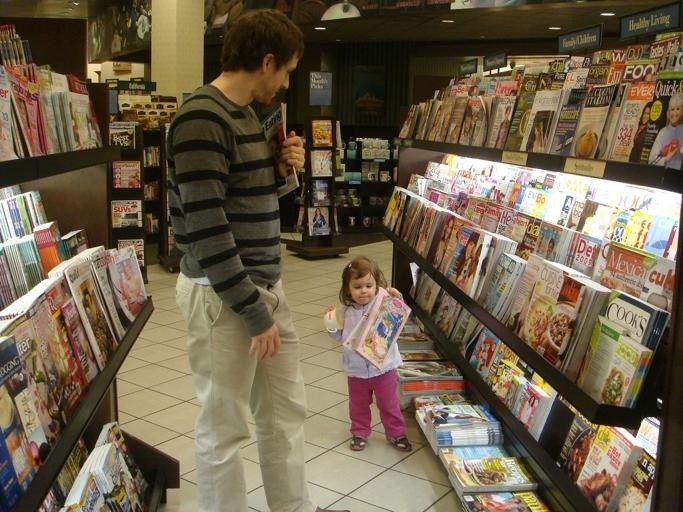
[315,505,350,512]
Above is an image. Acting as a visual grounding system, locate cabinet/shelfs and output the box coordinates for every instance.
[384,0,683,511]
[1,25,180,512]
[280,114,383,260]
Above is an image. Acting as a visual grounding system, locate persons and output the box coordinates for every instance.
[166,7,351,510]
[322,252,413,453]
[86,1,152,58]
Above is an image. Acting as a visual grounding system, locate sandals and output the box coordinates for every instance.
[391,435,412,451]
[350,436,366,450]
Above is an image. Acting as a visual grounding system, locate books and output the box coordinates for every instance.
[291,117,344,237]
[260,102,300,199]
[342,286,412,371]
